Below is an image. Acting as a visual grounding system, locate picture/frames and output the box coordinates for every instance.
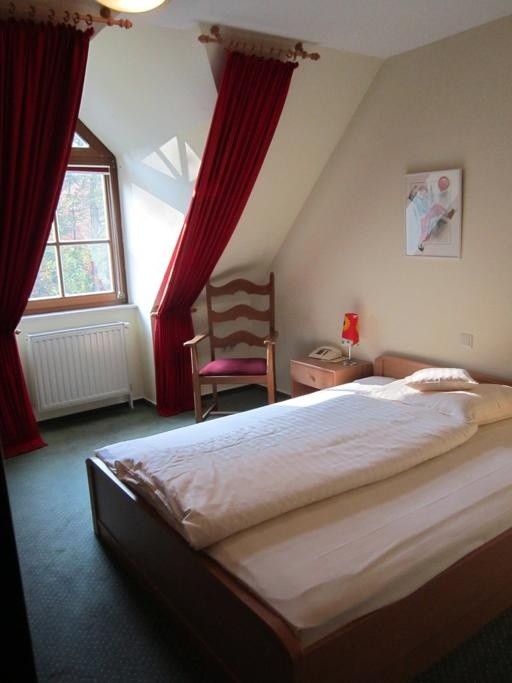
[403,166,464,258]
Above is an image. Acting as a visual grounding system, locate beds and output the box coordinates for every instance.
[85,354,512,683]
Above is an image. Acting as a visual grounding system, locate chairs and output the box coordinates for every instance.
[181,272,279,423]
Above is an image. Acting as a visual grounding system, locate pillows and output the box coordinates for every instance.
[403,365,476,390]
[379,374,512,424]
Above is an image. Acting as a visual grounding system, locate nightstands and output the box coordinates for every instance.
[289,356,372,398]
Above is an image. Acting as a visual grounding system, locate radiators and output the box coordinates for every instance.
[27,322,136,418]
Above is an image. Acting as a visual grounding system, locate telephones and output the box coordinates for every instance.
[308,346,342,361]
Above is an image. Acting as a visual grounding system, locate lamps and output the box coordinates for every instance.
[340,312,360,368]
